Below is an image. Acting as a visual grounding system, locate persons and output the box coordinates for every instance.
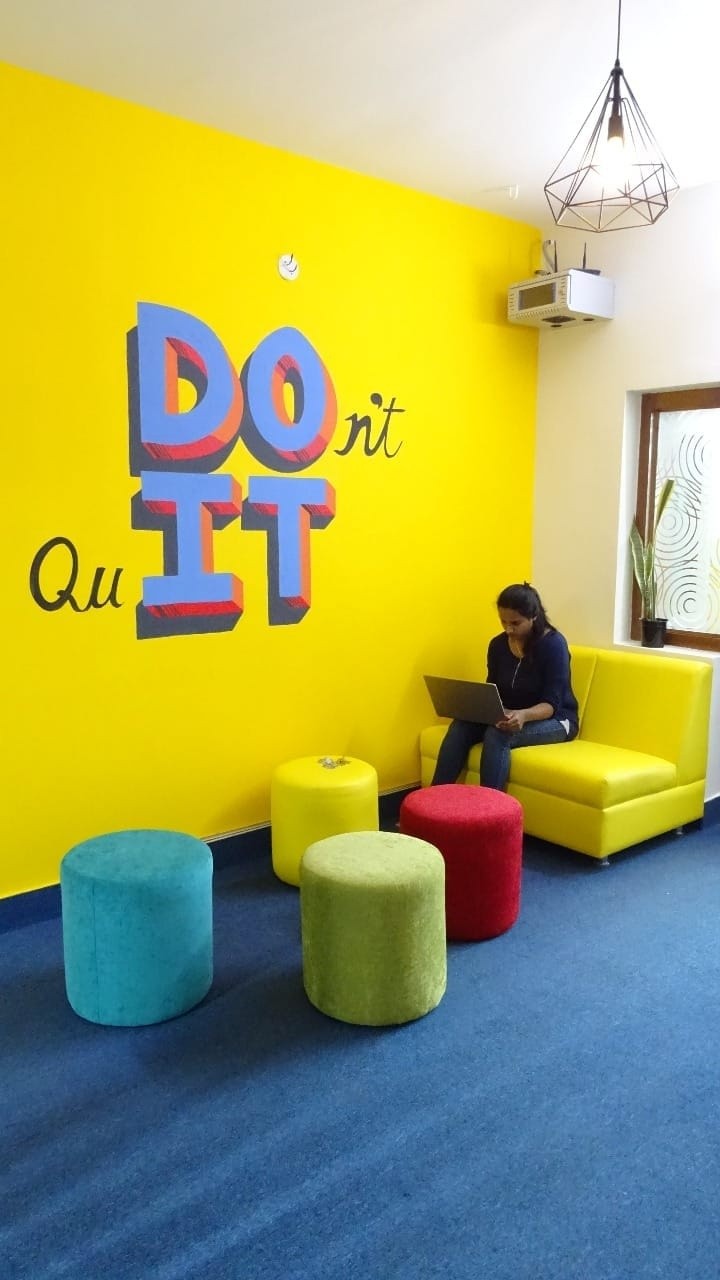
[431,580,580,793]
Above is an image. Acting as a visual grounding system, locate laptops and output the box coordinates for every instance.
[423,675,517,724]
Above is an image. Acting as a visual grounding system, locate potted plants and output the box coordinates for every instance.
[628,479,677,648]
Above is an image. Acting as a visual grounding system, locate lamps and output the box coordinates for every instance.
[544,0,681,233]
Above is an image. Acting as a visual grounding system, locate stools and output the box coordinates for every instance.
[300,831,447,1027]
[271,756,379,891]
[400,783,524,943]
[58,830,214,1027]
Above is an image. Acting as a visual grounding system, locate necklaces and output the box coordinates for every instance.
[516,639,523,658]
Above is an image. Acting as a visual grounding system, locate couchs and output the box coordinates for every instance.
[417,646,713,871]
